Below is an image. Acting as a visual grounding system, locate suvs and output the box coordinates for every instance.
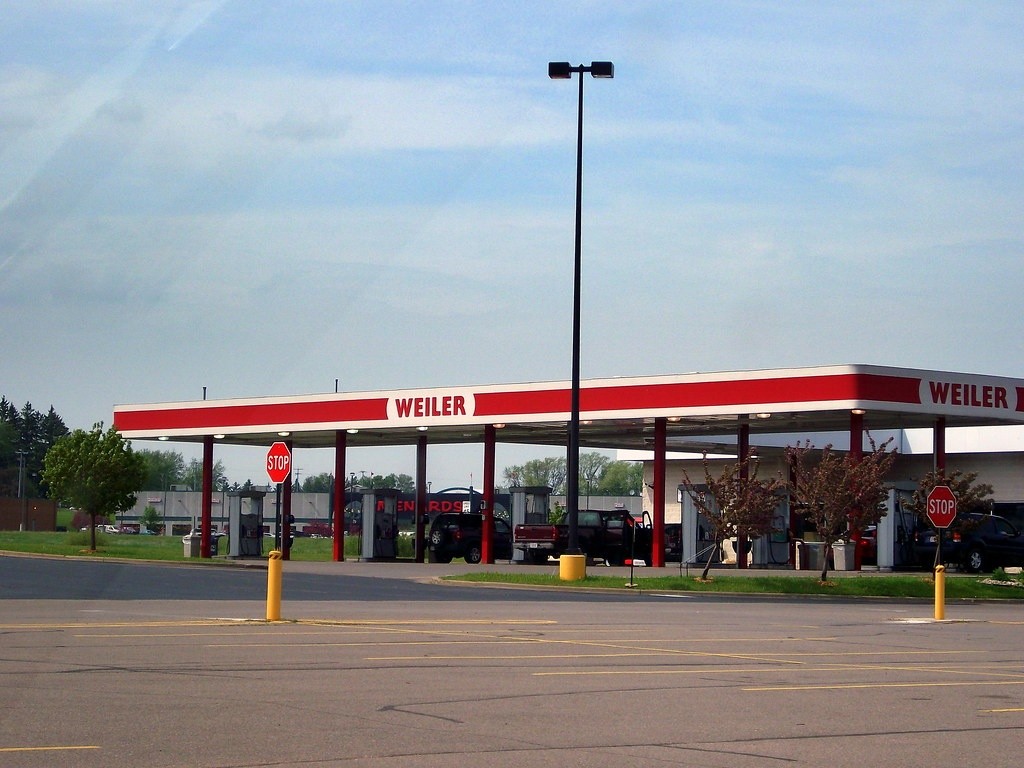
[428,511,513,564]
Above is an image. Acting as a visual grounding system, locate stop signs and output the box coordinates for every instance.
[928,486,958,530]
[266,442,290,484]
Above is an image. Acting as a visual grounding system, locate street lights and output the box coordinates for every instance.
[549,58,615,579]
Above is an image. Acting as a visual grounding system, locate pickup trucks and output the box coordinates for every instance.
[515,509,652,567]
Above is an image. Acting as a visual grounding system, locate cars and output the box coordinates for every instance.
[79,523,136,534]
[912,512,1024,572]
